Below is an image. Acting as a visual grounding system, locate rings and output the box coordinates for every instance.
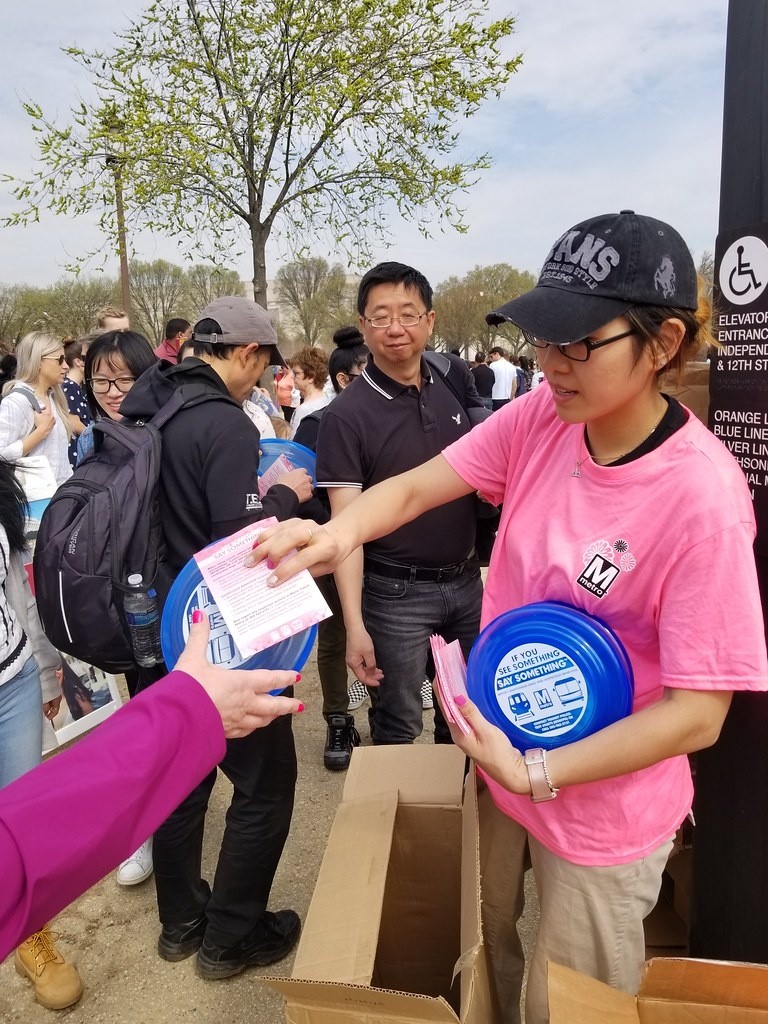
[307,529,313,544]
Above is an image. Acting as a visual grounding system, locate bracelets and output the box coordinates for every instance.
[525,748,560,802]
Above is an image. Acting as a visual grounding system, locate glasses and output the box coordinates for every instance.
[292,368,306,376]
[87,376,136,393]
[363,309,429,327]
[491,351,498,358]
[41,355,67,365]
[520,328,640,362]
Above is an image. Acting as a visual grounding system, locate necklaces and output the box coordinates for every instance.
[571,426,657,478]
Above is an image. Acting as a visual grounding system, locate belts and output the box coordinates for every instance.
[363,546,474,582]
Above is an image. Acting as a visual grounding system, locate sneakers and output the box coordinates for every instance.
[196,909,301,980]
[116,835,152,885]
[323,714,361,771]
[158,913,208,963]
[14,922,84,1009]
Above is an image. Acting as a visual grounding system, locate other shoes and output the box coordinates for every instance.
[420,674,434,708]
[347,678,371,710]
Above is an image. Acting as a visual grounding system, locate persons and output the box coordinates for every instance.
[0,457,84,1010]
[315,262,484,745]
[293,328,371,773]
[154,318,337,439]
[452,346,544,411]
[0,305,129,487]
[0,610,304,966]
[245,208,768,1024]
[117,297,316,982]
[77,329,159,885]
[48,653,93,732]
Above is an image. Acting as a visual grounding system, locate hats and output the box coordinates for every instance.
[484,208,697,346]
[191,296,289,370]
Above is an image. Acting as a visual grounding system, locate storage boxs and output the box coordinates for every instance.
[546,958,768,1024]
[247,743,494,1024]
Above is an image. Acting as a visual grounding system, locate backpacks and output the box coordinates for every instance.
[33,383,244,673]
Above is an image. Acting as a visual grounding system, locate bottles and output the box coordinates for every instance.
[123,574,166,667]
